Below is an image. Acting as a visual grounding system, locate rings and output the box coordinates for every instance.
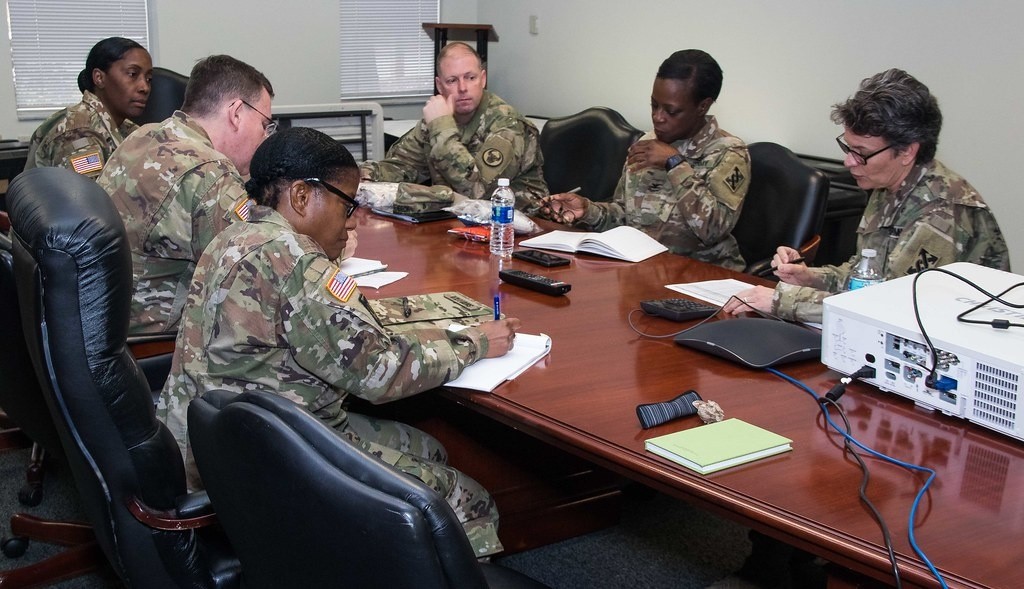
[743,298,747,303]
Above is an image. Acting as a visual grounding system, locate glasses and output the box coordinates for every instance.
[298,175,360,217]
[836,129,902,166]
[229,98,277,137]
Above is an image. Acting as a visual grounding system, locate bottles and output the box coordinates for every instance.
[847,249,887,292]
[489,178,515,256]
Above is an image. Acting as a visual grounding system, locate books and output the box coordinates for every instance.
[371,208,458,223]
[433,323,552,396]
[334,257,387,281]
[645,417,796,476]
[517,225,669,263]
[803,321,822,331]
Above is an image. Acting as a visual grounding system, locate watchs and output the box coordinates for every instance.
[665,153,688,173]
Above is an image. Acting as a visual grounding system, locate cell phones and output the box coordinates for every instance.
[512,249,570,267]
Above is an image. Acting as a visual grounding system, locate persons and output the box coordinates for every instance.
[721,67,1011,319]
[357,41,550,220]
[95,54,277,342]
[25,38,155,181]
[154,127,521,568]
[538,49,751,271]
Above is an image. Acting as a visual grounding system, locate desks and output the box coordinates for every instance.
[324,191,1024,589]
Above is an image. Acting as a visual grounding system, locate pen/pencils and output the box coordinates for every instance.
[494,295,500,321]
[532,187,582,212]
[403,295,411,318]
[758,257,806,278]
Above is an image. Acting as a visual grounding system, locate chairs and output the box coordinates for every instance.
[0,165,240,589]
[0,211,177,508]
[538,104,646,203]
[188,387,548,589]
[733,142,831,280]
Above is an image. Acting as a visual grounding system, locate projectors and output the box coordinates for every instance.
[820,262,1024,442]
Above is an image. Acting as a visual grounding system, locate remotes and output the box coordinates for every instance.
[498,269,571,296]
